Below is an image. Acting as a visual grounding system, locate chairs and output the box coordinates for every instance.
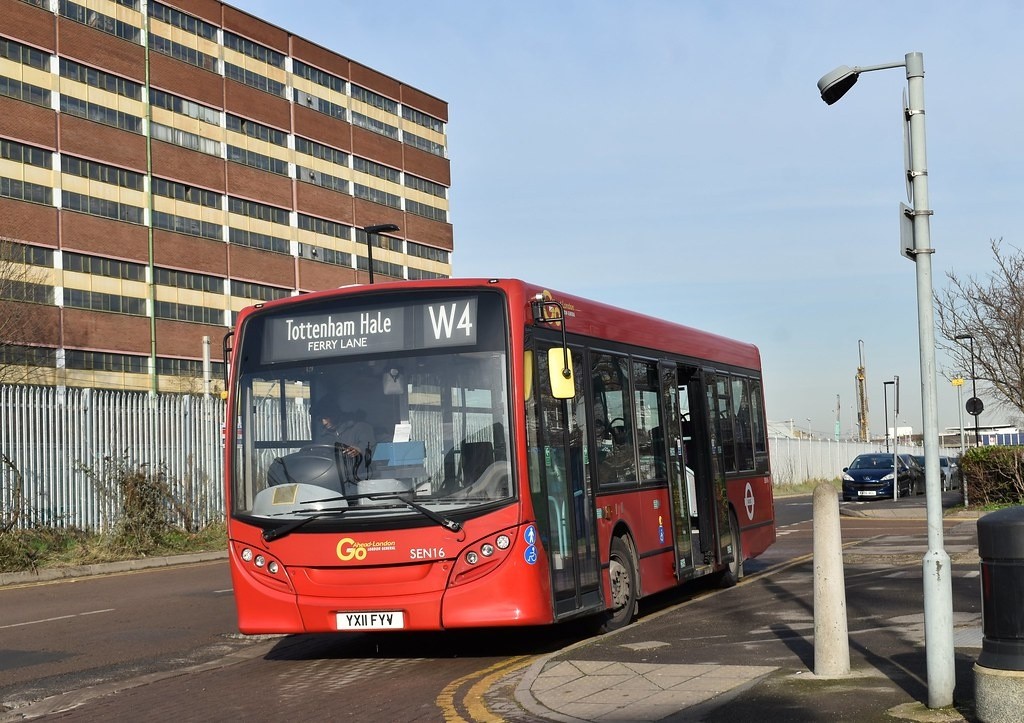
[603,411,757,473]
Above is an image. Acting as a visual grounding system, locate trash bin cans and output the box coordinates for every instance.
[975,505,1024,672]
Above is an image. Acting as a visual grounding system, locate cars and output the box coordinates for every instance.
[841,452,959,501]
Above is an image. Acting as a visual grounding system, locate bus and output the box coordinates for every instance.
[219,279,779,635]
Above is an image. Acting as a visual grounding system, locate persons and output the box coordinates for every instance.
[308,396,377,474]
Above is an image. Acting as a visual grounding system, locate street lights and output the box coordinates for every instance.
[816,51,960,709]
[362,221,399,284]
[954,334,983,446]
